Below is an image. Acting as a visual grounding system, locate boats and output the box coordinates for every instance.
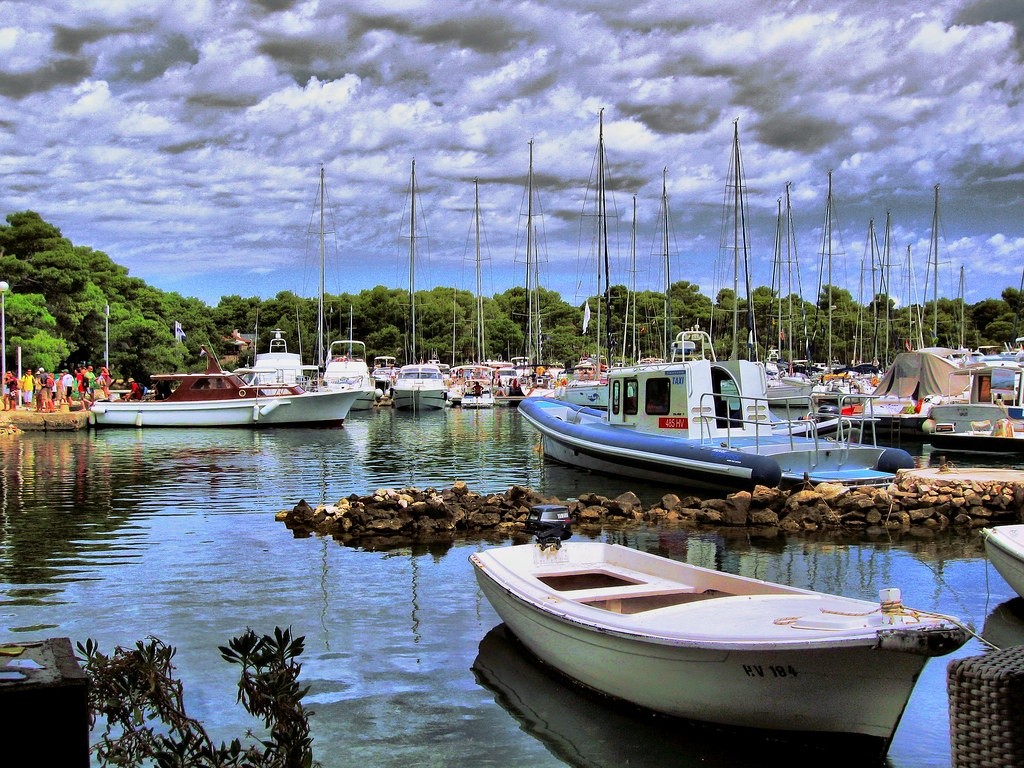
[90,343,364,427]
[515,321,916,491]
[467,503,975,738]
[978,523,1023,610]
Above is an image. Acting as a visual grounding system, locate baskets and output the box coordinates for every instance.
[946,646,1023,768]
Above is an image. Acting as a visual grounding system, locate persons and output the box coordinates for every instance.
[57,370,73,407]
[99,366,110,402]
[75,365,98,410]
[122,378,140,401]
[451,368,534,399]
[34,367,57,413]
[389,366,397,386]
[2,370,17,411]
[20,369,36,407]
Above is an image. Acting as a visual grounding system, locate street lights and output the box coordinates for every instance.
[0,282,10,399]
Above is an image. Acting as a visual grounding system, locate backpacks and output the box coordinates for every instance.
[80,374,89,387]
[46,377,54,388]
[13,376,23,389]
[137,382,147,396]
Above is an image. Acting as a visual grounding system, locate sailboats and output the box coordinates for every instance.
[239,107,1024,454]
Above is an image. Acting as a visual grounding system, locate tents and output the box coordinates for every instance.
[872,351,970,399]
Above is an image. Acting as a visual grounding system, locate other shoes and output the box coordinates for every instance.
[88,402,92,407]
[79,409,86,412]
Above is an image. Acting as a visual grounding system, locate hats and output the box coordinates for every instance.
[39,367,44,373]
[128,378,134,382]
[36,371,40,375]
[62,369,69,373]
